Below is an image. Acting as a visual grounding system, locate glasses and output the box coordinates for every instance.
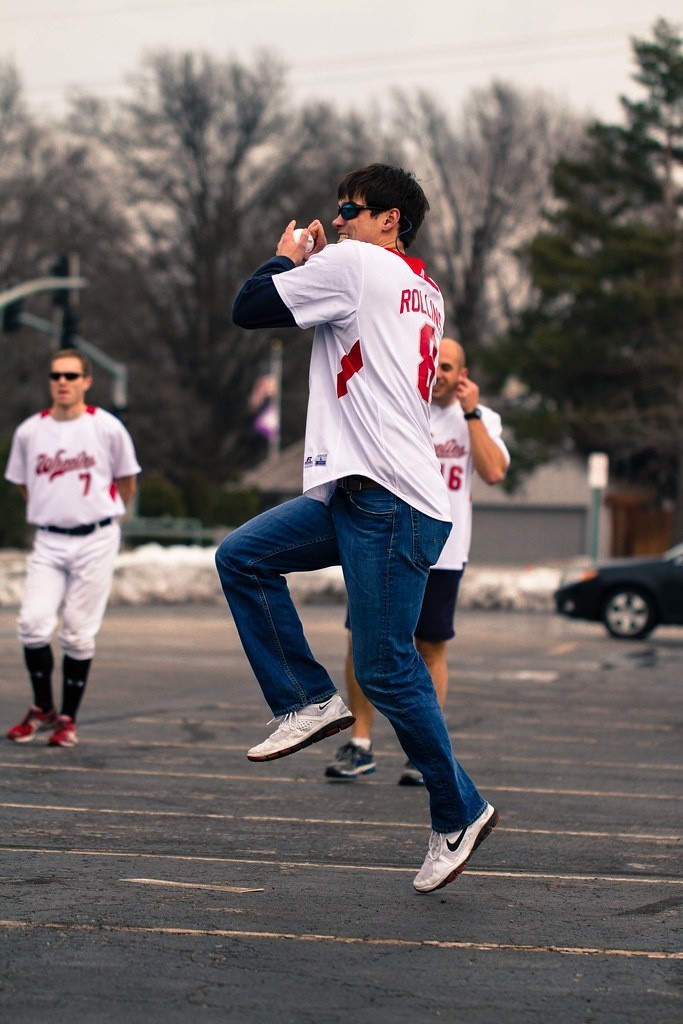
[48,371,84,380]
[337,201,388,221]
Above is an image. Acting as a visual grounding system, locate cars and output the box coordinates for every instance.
[551,540,683,642]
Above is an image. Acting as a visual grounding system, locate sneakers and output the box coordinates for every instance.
[247,692,356,762]
[7,702,58,744]
[48,714,78,748]
[326,742,376,779]
[412,799,499,894]
[399,754,426,786]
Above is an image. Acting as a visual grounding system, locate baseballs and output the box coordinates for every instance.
[293,228,315,252]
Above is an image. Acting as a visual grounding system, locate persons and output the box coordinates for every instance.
[319,335,512,792]
[3,349,143,749]
[213,163,505,895]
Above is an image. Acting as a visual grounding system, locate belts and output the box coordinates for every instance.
[41,518,110,536]
[337,477,382,491]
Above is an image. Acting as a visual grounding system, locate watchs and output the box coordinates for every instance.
[463,408,482,421]
